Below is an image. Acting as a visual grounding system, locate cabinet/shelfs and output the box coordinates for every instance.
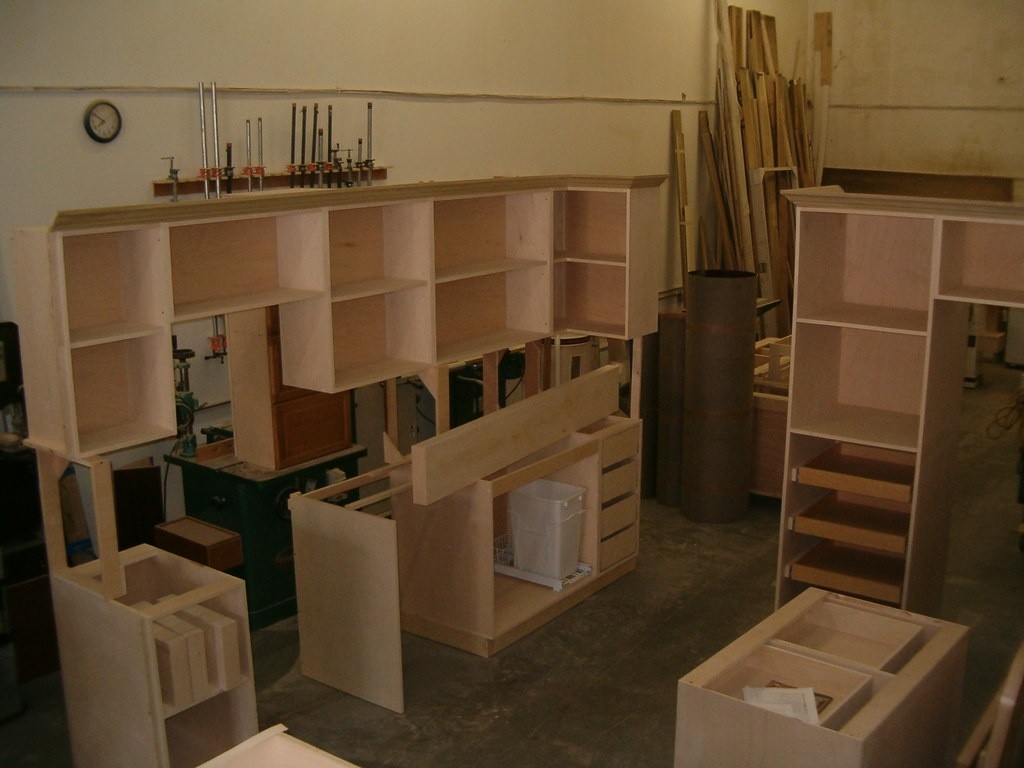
[12,170,663,471]
[776,185,1024,618]
[597,422,638,577]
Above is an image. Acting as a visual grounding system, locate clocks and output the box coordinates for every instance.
[83,98,122,143]
